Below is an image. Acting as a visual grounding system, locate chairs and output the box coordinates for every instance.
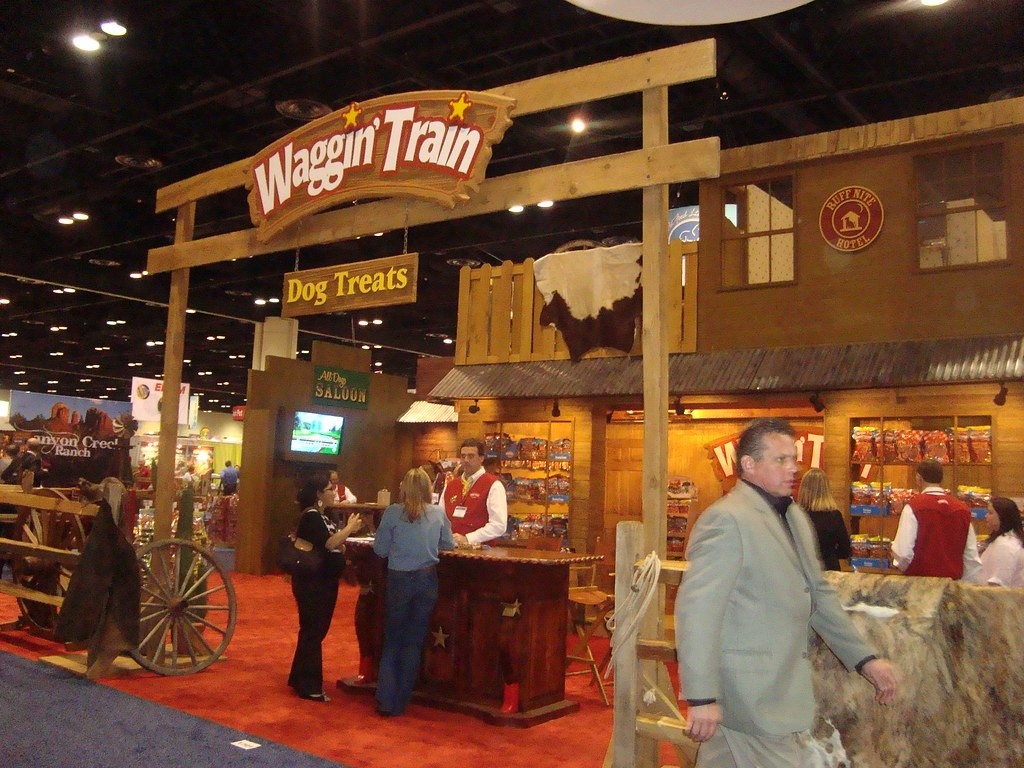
[559,565,617,707]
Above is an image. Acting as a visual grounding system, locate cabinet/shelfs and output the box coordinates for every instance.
[849,415,996,582]
[481,421,573,549]
[666,500,701,560]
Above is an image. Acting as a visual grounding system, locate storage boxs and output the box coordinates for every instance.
[203,545,234,571]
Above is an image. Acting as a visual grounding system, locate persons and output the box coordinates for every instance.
[234,464,239,471]
[421,465,435,484]
[979,497,1024,587]
[674,416,900,768]
[133,457,151,490]
[0,443,20,483]
[795,468,852,571]
[336,550,385,693]
[0,437,42,581]
[482,457,497,473]
[288,474,362,702]
[439,438,507,547]
[891,459,983,580]
[328,470,358,526]
[217,460,239,495]
[17,466,53,629]
[373,468,455,716]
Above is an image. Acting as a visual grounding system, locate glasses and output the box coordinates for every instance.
[322,486,335,492]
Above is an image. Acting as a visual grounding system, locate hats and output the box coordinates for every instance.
[917,459,943,483]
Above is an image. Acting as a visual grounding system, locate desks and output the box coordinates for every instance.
[341,537,604,725]
[323,501,387,535]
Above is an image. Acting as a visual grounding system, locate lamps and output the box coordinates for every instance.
[673,394,683,415]
[468,399,480,414]
[273,82,334,123]
[551,398,560,417]
[113,139,161,168]
[992,381,1009,406]
[808,390,827,414]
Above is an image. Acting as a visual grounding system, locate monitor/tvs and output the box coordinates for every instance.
[290,412,344,456]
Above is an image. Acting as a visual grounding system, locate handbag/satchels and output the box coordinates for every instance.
[287,507,325,562]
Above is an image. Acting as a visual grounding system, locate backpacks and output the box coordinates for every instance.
[7,453,33,485]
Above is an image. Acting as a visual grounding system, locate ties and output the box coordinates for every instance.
[461,478,474,499]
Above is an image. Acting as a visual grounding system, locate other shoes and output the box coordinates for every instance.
[299,690,331,702]
[287,679,326,694]
[375,702,393,713]
